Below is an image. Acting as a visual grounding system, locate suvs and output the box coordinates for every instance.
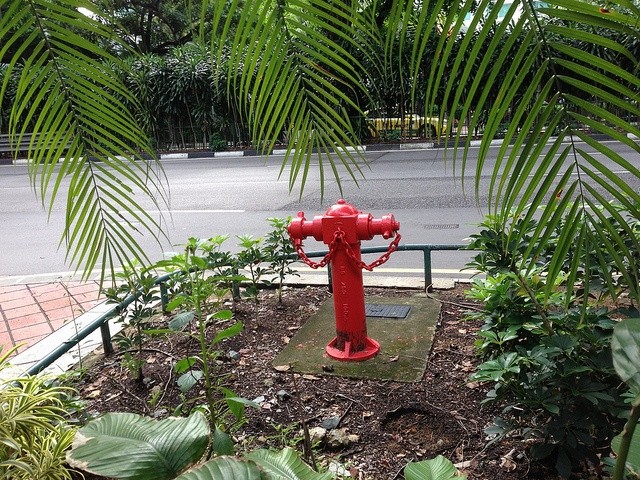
[361,105,448,140]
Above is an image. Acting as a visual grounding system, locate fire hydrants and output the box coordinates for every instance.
[288,199,402,361]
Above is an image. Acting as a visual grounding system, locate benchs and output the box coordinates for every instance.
[0,131,75,163]
